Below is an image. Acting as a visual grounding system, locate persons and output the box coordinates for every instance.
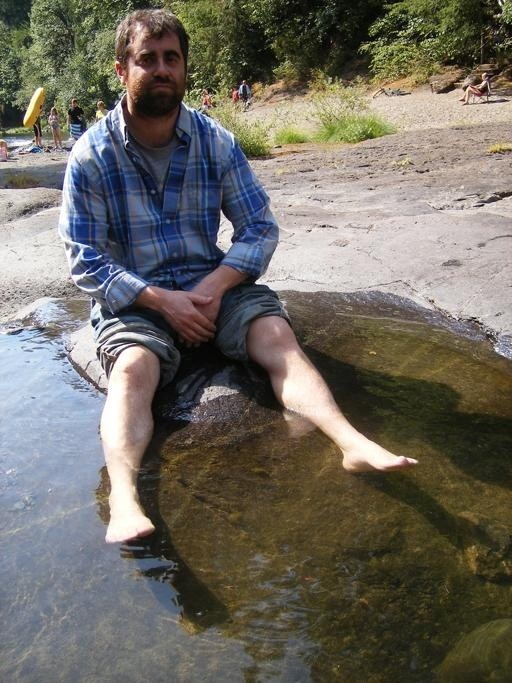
[68,98,86,139]
[95,99,110,122]
[0,138,10,161]
[239,79,252,104]
[201,88,212,111]
[230,85,240,107]
[48,106,63,150]
[33,104,45,147]
[457,71,491,107]
[58,6,420,546]
